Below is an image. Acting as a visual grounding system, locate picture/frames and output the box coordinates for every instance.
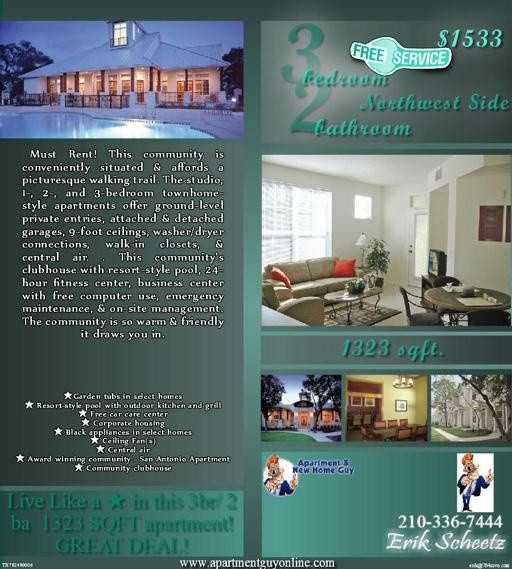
[505,205,511,242]
[478,205,503,241]
[350,397,362,407]
[395,400,407,412]
[364,397,376,407]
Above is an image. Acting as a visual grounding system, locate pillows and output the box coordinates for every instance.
[271,267,292,288]
[334,259,357,277]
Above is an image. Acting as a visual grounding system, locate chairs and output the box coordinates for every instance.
[347,412,427,442]
[399,286,444,326]
[261,281,325,327]
[468,310,511,326]
[433,276,463,326]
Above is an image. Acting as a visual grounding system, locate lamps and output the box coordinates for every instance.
[392,375,414,389]
[355,232,369,269]
[217,68,220,71]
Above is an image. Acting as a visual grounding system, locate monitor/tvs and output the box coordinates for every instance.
[428,249,447,279]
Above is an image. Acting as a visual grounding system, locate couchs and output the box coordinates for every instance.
[263,257,360,307]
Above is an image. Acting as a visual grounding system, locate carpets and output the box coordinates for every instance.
[324,301,402,326]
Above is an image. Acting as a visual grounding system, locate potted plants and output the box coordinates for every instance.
[364,236,391,288]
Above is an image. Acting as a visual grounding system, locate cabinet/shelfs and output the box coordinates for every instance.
[419,275,437,312]
